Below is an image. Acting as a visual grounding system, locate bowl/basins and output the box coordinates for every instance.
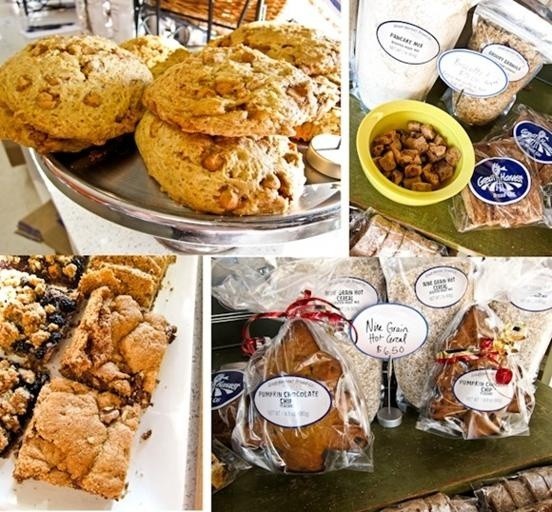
[356,102,475,207]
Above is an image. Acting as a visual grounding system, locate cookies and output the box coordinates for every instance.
[0,19,341,218]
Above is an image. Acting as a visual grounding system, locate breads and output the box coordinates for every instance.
[212,256,552,492]
[0,256,178,502]
[458,143,543,226]
[380,466,552,512]
[505,109,552,187]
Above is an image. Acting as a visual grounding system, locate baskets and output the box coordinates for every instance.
[150,0,285,30]
